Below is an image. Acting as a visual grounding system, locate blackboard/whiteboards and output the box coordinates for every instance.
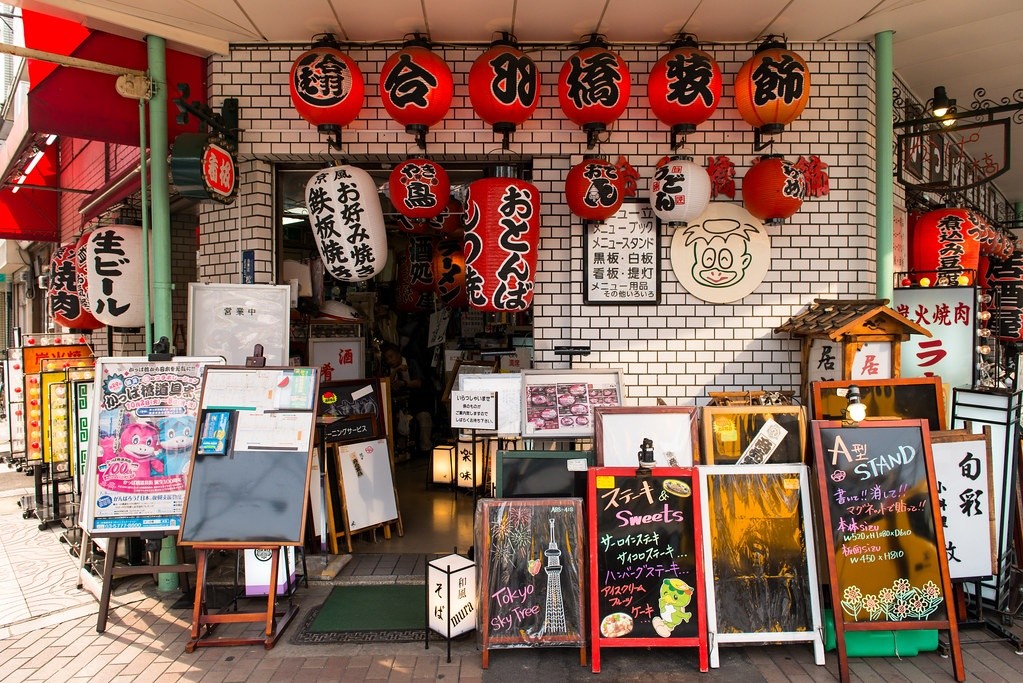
[811,419,955,628]
[315,377,393,441]
[176,363,322,548]
[936,426,999,580]
[321,413,377,442]
[703,405,807,465]
[588,466,707,648]
[337,439,399,535]
[592,405,701,467]
[473,496,586,646]
[494,450,595,497]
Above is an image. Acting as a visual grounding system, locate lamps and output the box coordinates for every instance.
[489,435,522,499]
[840,383,867,427]
[943,98,957,127]
[426,445,456,492]
[424,546,478,664]
[932,86,948,118]
[454,436,485,500]
[635,437,657,480]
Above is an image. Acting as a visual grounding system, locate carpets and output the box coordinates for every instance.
[304,585,426,633]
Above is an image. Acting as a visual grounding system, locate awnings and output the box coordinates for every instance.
[1,6,207,243]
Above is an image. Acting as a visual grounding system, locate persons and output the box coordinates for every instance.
[382,342,434,459]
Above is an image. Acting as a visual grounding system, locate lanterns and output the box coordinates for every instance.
[647,39,723,135]
[558,42,631,132]
[304,158,388,283]
[468,40,540,134]
[981,250,1023,343]
[980,220,1014,259]
[460,162,541,314]
[734,43,810,135]
[45,219,153,334]
[910,208,980,286]
[380,40,454,136]
[390,191,471,316]
[742,153,806,225]
[289,42,364,136]
[564,154,625,226]
[389,155,450,222]
[649,157,712,228]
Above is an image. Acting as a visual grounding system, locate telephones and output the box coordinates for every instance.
[811,376,946,431]
